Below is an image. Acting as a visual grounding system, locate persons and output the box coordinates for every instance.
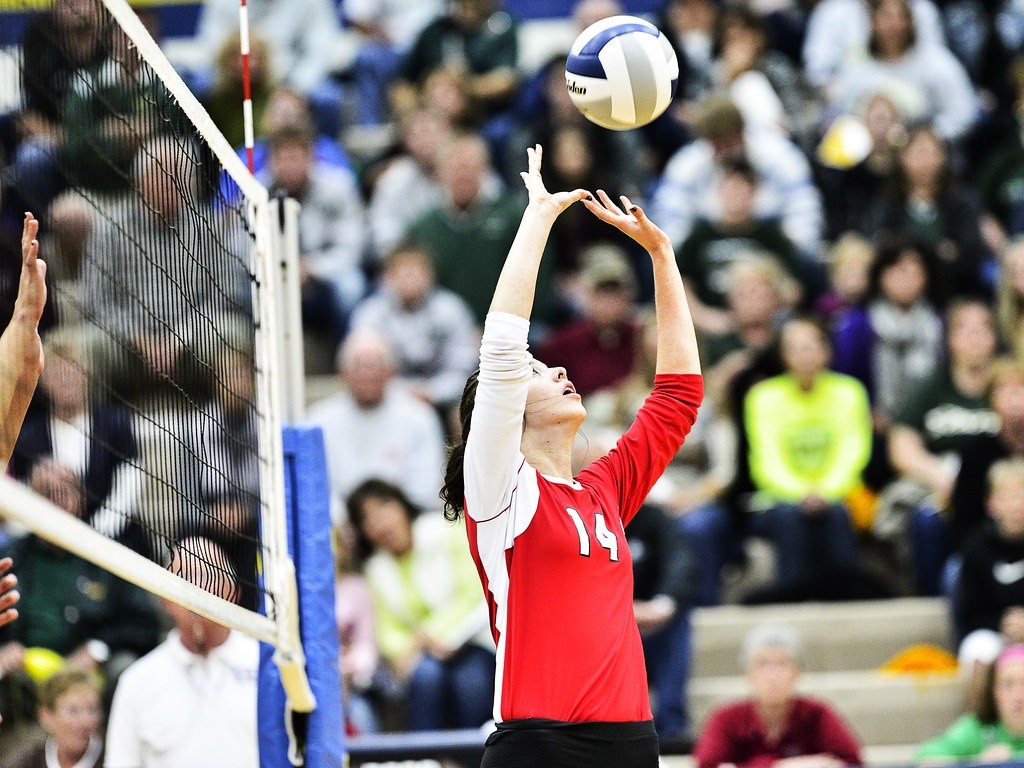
[1,2,1022,768]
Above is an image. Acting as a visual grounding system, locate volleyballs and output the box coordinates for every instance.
[562,13,679,131]
